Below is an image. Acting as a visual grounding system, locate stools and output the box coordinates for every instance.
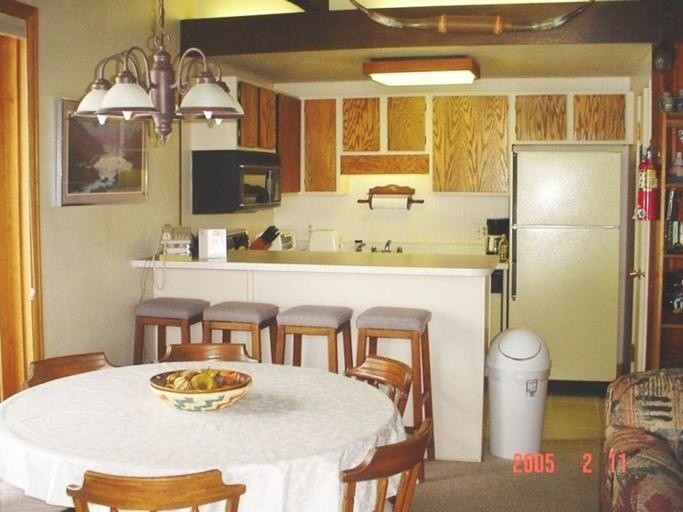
[356,306,435,482]
[132,297,210,364]
[203,301,278,363]
[273,305,353,379]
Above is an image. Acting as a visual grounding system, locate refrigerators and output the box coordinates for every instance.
[506,144,635,384]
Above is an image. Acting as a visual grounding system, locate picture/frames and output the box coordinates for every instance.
[56,95,148,207]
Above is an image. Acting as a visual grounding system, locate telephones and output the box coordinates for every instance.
[153,224,191,247]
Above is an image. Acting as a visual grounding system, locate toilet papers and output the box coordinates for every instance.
[371,195,409,209]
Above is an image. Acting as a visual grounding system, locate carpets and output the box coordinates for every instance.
[403,437,604,512]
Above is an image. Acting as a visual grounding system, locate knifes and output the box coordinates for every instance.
[260,226,280,243]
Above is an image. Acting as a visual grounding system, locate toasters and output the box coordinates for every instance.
[308,230,339,252]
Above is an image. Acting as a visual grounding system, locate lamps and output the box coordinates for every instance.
[67,0,246,144]
[363,56,481,86]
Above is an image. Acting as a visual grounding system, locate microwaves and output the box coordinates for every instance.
[192,150,282,215]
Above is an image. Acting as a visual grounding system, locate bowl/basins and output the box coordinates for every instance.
[147,369,253,413]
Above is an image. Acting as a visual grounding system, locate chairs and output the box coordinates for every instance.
[344,355,414,417]
[66,469,246,512]
[340,418,434,512]
[157,343,260,364]
[23,351,120,390]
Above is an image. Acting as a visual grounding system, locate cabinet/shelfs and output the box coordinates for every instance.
[647,111,683,371]
[277,93,302,193]
[304,98,336,192]
[190,81,277,152]
[432,96,509,194]
[340,96,430,176]
[515,94,625,143]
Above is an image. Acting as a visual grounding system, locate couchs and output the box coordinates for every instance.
[600,369,683,511]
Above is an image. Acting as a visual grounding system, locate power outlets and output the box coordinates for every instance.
[476,224,487,238]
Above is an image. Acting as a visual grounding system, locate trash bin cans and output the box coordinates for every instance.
[485,328,552,461]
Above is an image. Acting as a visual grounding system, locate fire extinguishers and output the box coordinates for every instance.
[637,139,661,221]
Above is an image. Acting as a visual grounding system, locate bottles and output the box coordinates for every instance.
[498,234,508,263]
[667,152,683,182]
